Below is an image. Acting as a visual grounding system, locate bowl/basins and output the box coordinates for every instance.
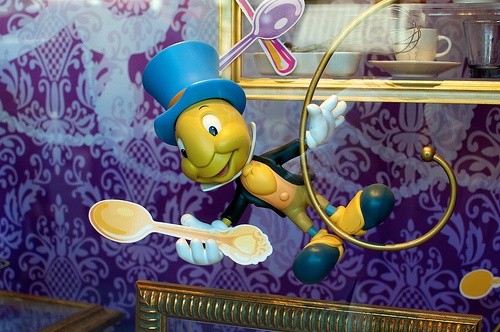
[254,50,283,74]
[288,50,320,76]
[315,52,363,78]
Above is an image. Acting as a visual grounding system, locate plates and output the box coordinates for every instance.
[368,58,463,79]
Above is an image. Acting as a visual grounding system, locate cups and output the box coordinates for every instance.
[392,27,452,60]
[463,20,500,78]
